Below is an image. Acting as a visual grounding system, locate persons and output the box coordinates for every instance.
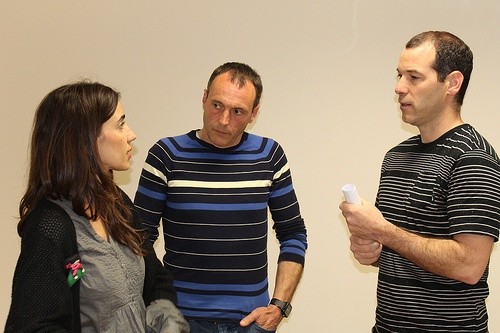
[3,77,193,333]
[134,62,308,333]
[339,29,500,333]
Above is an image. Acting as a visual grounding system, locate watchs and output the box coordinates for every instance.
[269,298,293,319]
